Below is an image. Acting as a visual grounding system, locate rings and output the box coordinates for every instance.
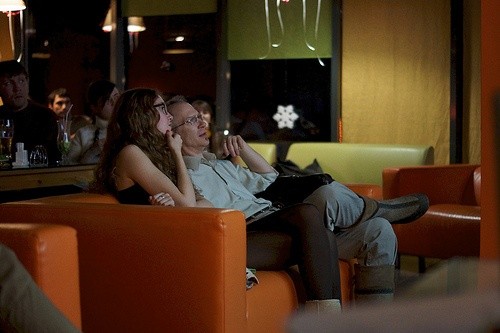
[223,141,226,144]
[160,196,165,198]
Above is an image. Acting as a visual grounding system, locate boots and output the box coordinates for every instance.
[352,262,396,305]
[337,192,430,231]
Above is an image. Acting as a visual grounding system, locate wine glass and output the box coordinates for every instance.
[56,120,72,165]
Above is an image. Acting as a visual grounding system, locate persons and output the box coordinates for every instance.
[162,93,432,306]
[0,59,291,202]
[98,88,347,314]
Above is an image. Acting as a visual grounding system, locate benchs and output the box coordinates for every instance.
[0,141,481,333]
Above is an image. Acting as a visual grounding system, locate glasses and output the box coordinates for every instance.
[171,113,205,132]
[153,102,168,115]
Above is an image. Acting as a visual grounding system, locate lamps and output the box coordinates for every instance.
[102,7,145,33]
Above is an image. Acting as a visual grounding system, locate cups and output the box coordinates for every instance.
[30,147,48,167]
[0,119,14,170]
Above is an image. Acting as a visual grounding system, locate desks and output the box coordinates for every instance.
[0,164,98,192]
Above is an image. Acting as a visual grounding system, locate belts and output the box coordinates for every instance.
[246,205,280,225]
